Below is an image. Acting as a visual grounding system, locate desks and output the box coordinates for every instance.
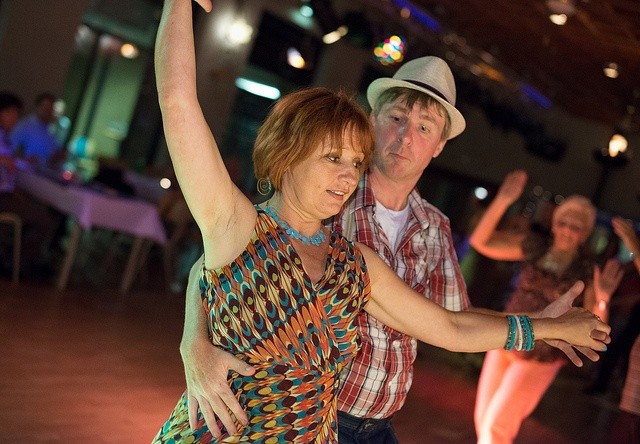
[17,169,168,295]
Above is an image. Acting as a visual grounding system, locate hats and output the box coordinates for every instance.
[367,56,466,140]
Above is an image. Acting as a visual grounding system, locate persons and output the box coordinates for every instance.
[468,169,627,444]
[0,92,60,286]
[9,92,73,262]
[180,55,608,444]
[151,0,610,444]
[611,212,640,444]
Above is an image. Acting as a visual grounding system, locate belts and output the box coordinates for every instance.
[338,414,391,433]
